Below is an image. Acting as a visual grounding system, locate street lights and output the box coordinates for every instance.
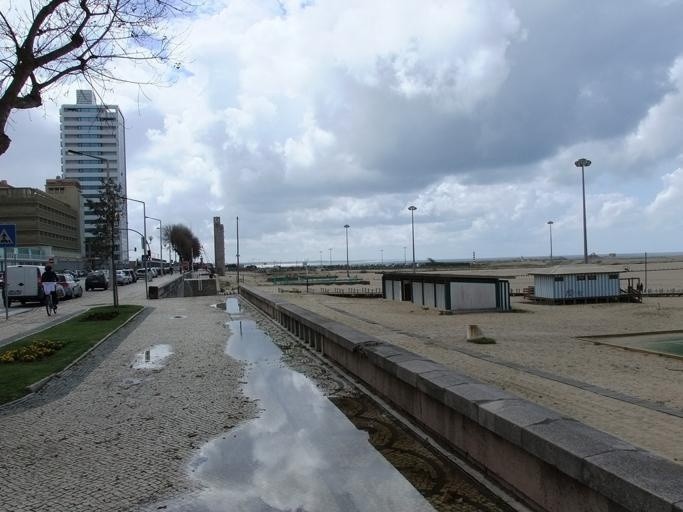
[68,150,118,307]
[575,159,591,264]
[408,206,417,272]
[547,221,553,260]
[344,224,350,277]
[115,195,172,299]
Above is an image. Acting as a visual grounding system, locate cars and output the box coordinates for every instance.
[86,266,168,291]
[55,273,82,301]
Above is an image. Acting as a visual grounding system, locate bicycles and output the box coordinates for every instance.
[46,281,57,316]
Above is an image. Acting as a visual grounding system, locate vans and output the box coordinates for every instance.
[2,265,46,308]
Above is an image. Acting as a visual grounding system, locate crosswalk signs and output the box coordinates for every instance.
[0,224,16,247]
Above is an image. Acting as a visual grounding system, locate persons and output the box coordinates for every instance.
[208,263,215,279]
[41,266,58,309]
